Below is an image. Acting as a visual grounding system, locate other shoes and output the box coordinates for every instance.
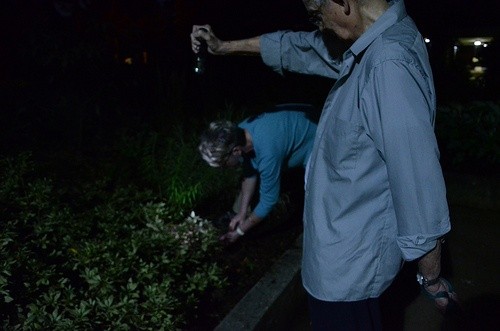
[272,223,303,255]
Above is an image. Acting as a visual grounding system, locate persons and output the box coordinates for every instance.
[198,109,319,245]
[191,0,459,331]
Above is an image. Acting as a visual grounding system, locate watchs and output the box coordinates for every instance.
[416,270,442,288]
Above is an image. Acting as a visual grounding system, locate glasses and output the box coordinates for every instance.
[308,5,325,27]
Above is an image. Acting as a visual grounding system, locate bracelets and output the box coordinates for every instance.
[236,226,247,236]
